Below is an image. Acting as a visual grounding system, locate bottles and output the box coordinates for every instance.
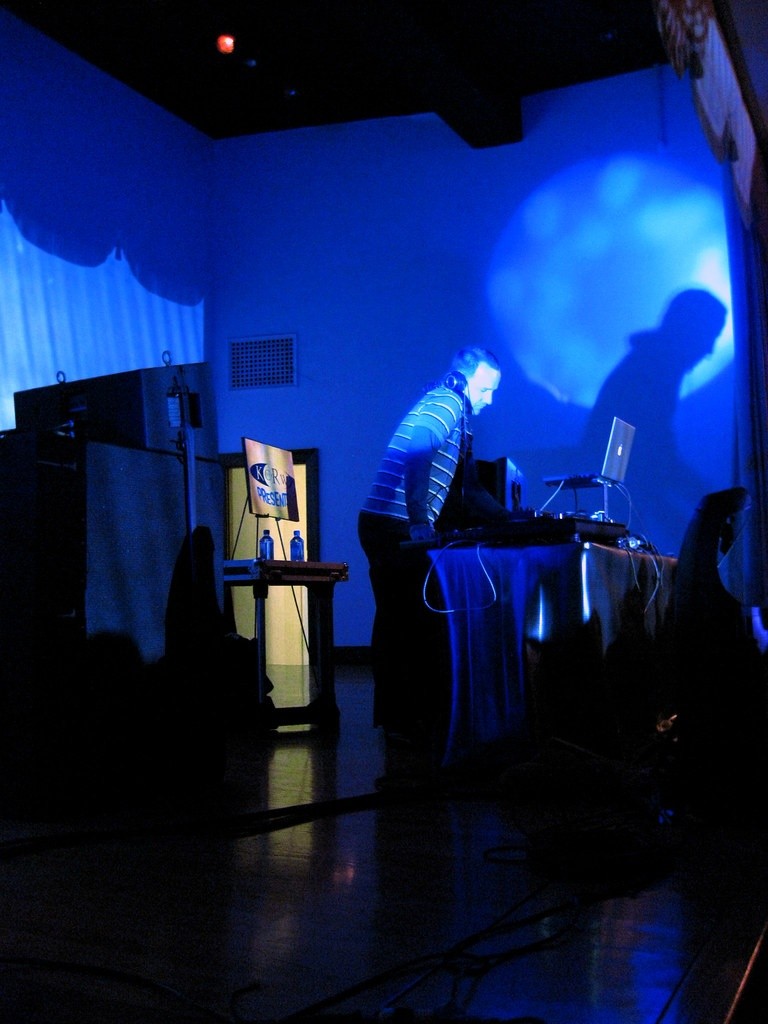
[259,530,274,561]
[290,530,304,561]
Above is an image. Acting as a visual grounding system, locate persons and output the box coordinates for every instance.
[356,343,510,797]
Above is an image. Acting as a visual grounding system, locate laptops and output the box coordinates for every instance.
[544,416,636,490]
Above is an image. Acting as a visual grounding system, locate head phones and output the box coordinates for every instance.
[441,370,467,389]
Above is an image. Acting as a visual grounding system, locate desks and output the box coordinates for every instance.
[225,557,352,739]
[428,540,680,798]
